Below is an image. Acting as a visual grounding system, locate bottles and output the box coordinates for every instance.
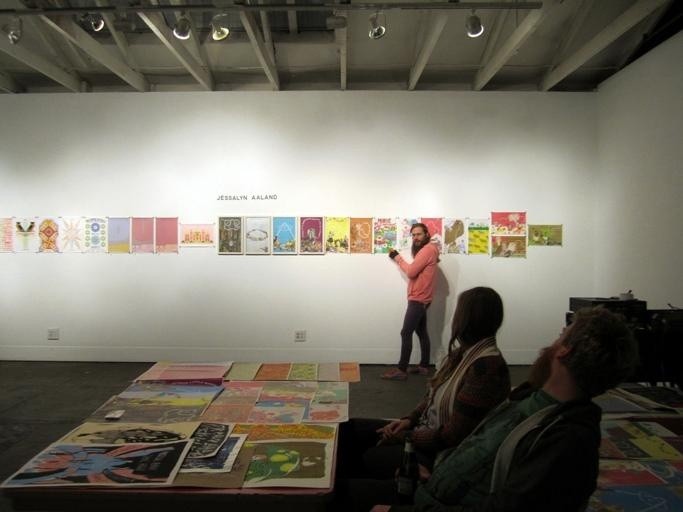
[392,437,420,505]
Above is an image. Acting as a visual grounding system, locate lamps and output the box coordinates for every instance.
[0,2,545,45]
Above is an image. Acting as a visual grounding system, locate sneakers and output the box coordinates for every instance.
[380,367,409,381]
[410,364,433,376]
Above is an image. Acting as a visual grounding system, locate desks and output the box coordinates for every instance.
[0,424,341,512]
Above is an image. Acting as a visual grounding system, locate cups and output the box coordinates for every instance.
[619,293,633,301]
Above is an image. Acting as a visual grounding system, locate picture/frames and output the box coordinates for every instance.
[216,216,243,255]
[272,216,298,255]
[298,216,325,255]
[243,216,271,256]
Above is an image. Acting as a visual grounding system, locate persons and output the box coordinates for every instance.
[378,223,441,380]
[326,304,641,512]
[334,286,511,480]
